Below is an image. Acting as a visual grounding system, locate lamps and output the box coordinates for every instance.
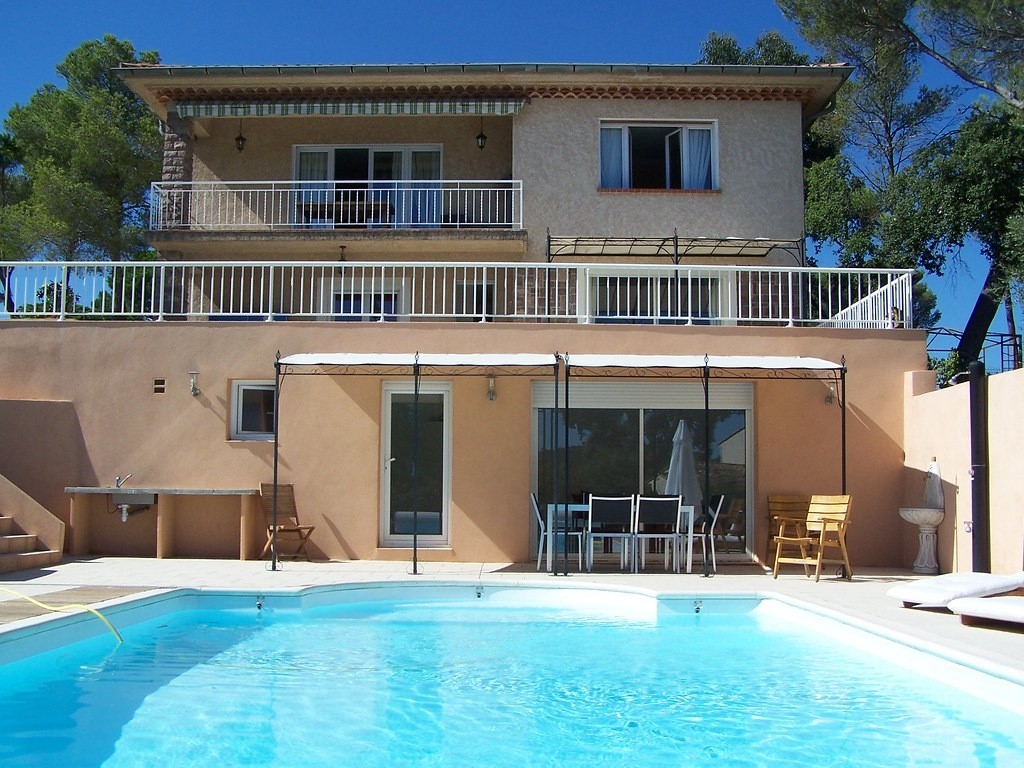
[235,118,246,152]
[824,380,838,406]
[188,370,200,396]
[475,116,487,150]
[485,374,498,401]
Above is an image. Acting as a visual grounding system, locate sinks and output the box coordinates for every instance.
[112,488,158,505]
[899,508,945,526]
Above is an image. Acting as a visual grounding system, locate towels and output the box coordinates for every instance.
[665,419,703,526]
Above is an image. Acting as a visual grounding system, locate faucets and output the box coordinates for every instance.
[115,474,132,488]
[923,472,932,481]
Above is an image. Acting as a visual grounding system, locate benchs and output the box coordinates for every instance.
[887,572,1024,625]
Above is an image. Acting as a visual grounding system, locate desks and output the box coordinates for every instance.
[546,504,695,574]
[295,201,394,229]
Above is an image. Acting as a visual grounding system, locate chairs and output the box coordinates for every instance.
[258,482,316,561]
[529,493,724,574]
[768,494,826,570]
[773,494,854,582]
